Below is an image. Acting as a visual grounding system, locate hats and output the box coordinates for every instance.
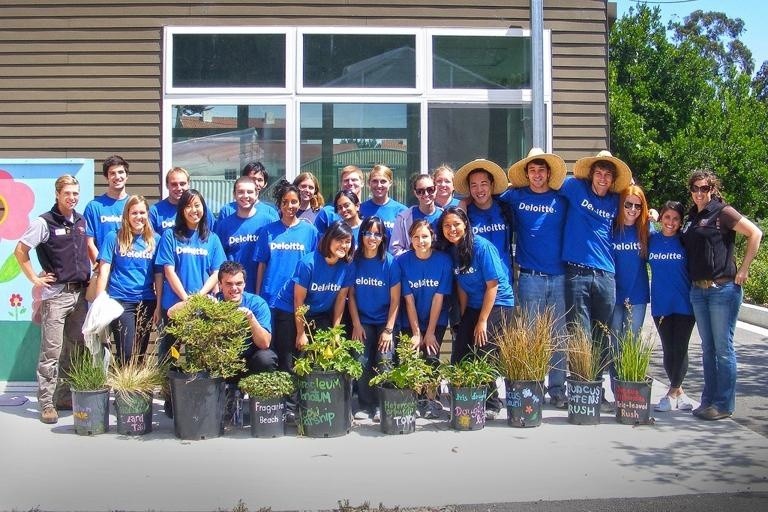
[452,157,509,195]
[573,149,632,193]
[508,148,568,190]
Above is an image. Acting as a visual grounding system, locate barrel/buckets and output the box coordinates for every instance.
[296,368,353,437]
[379,386,416,435]
[251,397,287,439]
[114,390,152,436]
[567,375,605,425]
[615,373,652,425]
[505,378,543,427]
[165,370,227,440]
[449,382,489,431]
[70,389,110,437]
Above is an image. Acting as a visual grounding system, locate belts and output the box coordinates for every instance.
[692,278,733,289]
[64,281,83,293]
[520,268,548,277]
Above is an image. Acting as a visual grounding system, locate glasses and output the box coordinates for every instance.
[690,184,711,194]
[414,186,436,197]
[625,201,643,210]
[361,231,382,238]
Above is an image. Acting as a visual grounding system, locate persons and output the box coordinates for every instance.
[94,194,160,366]
[213,175,282,295]
[166,259,280,418]
[155,187,228,401]
[218,160,282,219]
[147,166,216,236]
[82,153,134,276]
[13,172,91,425]
[252,158,518,418]
[611,183,654,399]
[557,149,663,414]
[457,147,569,410]
[677,171,763,420]
[644,199,697,411]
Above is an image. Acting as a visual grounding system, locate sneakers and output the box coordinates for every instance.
[55,399,72,411]
[425,398,441,419]
[485,408,498,420]
[226,387,243,419]
[653,393,735,421]
[547,390,569,408]
[373,407,380,422]
[354,407,370,420]
[41,406,59,424]
[600,388,614,413]
